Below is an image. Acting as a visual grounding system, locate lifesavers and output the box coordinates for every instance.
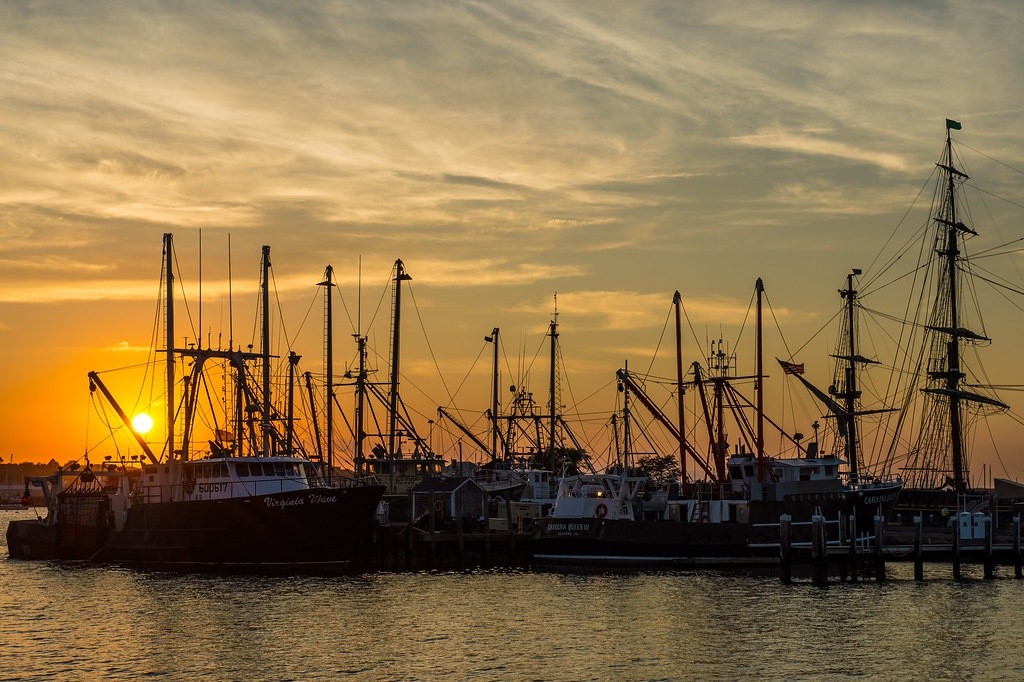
[701,515,710,524]
[772,474,779,482]
[941,509,949,516]
[434,500,444,512]
[595,503,608,518]
[643,492,651,501]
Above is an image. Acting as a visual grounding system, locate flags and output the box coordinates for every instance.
[779,361,805,375]
[217,430,232,442]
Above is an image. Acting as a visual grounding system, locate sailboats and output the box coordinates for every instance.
[7,117,1024,561]
